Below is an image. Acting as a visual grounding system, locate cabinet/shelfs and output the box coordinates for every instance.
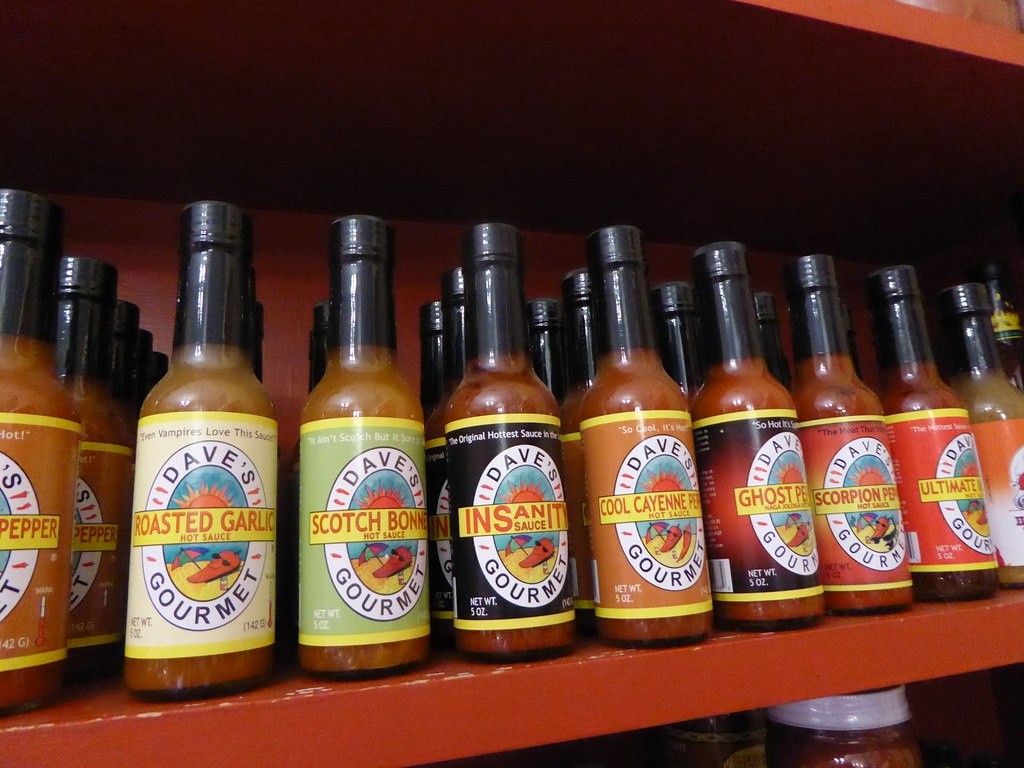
[0,0,1024,768]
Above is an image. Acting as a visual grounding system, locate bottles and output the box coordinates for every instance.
[0,189,1024,717]
